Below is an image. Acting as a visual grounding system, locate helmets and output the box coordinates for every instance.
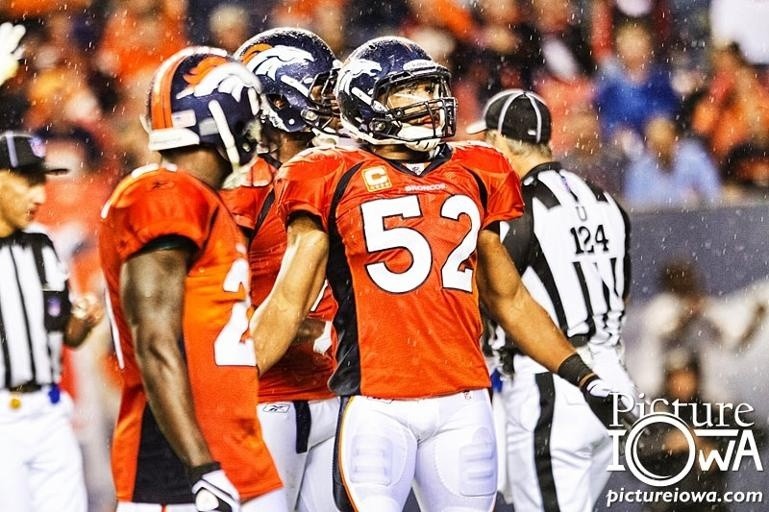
[147,26,457,154]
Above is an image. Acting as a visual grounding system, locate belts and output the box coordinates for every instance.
[10,384,41,393]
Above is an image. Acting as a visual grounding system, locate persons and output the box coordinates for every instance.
[1,1,769,512]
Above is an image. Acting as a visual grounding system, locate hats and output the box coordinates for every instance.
[464,88,550,147]
[0,130,69,178]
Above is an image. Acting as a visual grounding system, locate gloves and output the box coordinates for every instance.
[187,461,240,512]
[581,375,650,456]
